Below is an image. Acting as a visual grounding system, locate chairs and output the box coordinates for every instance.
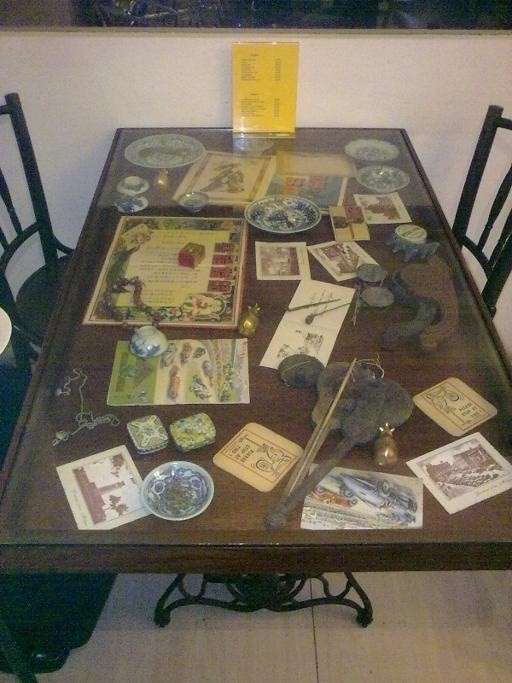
[450,104,512,320]
[0,91,77,371]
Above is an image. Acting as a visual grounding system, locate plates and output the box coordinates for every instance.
[115,175,150,196]
[243,194,323,236]
[354,164,411,195]
[112,191,148,215]
[140,459,216,523]
[344,138,400,164]
[123,133,206,170]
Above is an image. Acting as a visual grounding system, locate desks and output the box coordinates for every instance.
[0,127,512,627]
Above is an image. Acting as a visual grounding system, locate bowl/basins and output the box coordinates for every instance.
[176,191,209,215]
[128,325,169,360]
